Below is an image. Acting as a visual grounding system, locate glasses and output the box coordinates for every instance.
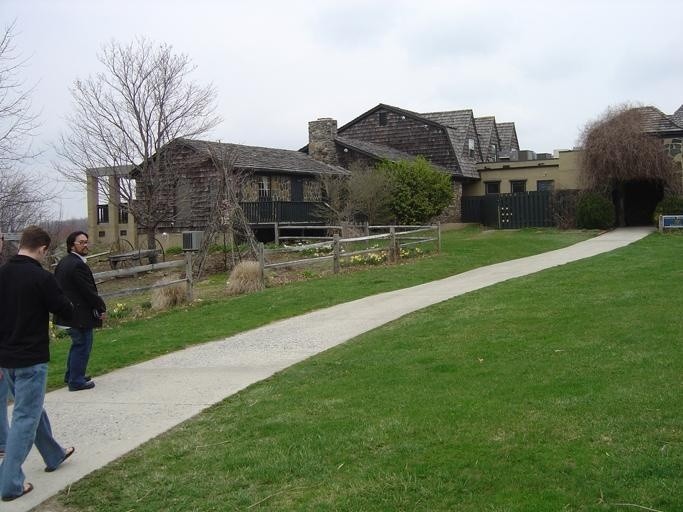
[74,240,85,247]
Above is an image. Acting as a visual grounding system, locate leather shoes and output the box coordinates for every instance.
[67,380,95,392]
[64,374,92,383]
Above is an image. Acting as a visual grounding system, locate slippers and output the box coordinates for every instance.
[1,482,33,502]
[44,445,75,473]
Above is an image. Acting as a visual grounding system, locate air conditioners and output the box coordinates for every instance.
[182,231,206,251]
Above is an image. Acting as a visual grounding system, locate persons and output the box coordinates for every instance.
[50,231,106,393]
[0,236,17,457]
[0,225,77,501]
